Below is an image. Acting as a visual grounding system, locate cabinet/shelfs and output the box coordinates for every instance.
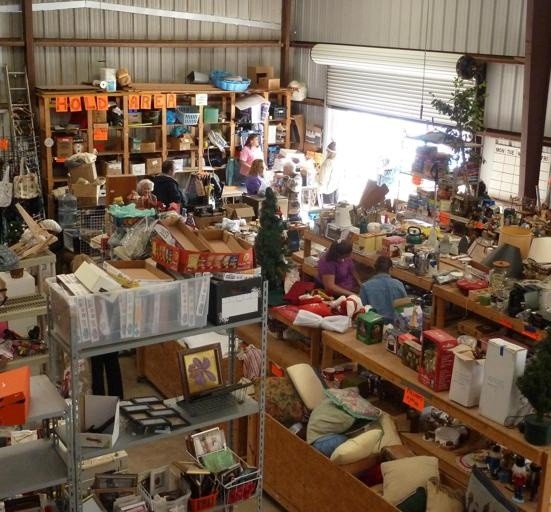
[0,259,76,512]
[46,273,265,511]
[39,87,290,221]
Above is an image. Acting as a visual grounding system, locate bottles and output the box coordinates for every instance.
[464,265,472,281]
[367,374,380,396]
[488,444,538,504]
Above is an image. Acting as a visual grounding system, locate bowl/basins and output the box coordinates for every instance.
[436,427,460,451]
[217,77,251,91]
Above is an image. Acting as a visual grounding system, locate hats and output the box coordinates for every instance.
[327,141,337,153]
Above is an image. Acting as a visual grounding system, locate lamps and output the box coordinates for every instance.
[525,236,551,267]
[481,242,522,278]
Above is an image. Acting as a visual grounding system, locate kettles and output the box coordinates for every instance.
[407,226,423,244]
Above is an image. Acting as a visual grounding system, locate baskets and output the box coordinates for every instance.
[175,105,200,125]
[189,479,256,512]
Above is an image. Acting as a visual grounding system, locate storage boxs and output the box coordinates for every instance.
[259,76,280,90]
[46,271,212,347]
[247,66,274,88]
[225,202,254,222]
[150,229,254,273]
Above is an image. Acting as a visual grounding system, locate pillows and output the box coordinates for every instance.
[380,455,440,506]
[287,362,324,408]
[253,376,303,420]
[330,428,383,464]
[306,398,353,445]
[426,479,467,511]
[323,386,380,419]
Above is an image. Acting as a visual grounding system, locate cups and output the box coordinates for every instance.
[321,366,344,382]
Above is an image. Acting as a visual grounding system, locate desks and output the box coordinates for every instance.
[321,327,550,512]
[435,282,551,353]
[294,227,434,312]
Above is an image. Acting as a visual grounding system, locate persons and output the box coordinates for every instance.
[136,178,158,202]
[278,163,302,217]
[239,132,269,184]
[89,349,124,400]
[317,239,363,300]
[315,140,340,204]
[361,255,410,321]
[155,159,186,207]
[247,158,266,193]
